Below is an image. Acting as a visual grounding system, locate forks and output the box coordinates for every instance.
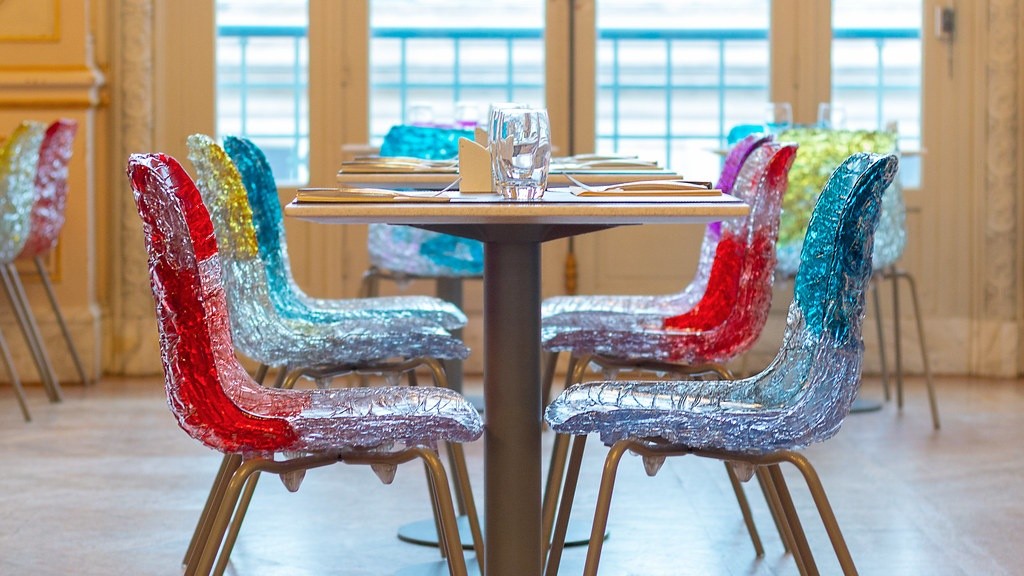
[296,152,462,203]
[549,149,709,192]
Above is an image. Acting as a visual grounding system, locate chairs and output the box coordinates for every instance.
[113,152,485,576]
[347,123,496,312]
[179,136,476,565]
[542,154,905,576]
[0,117,60,422]
[537,129,777,409]
[721,123,894,414]
[3,120,90,402]
[531,141,802,564]
[777,123,943,432]
[221,134,470,403]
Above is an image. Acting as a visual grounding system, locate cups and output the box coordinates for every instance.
[485,101,553,199]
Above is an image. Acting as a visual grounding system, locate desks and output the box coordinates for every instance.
[336,142,688,186]
[287,182,752,576]
